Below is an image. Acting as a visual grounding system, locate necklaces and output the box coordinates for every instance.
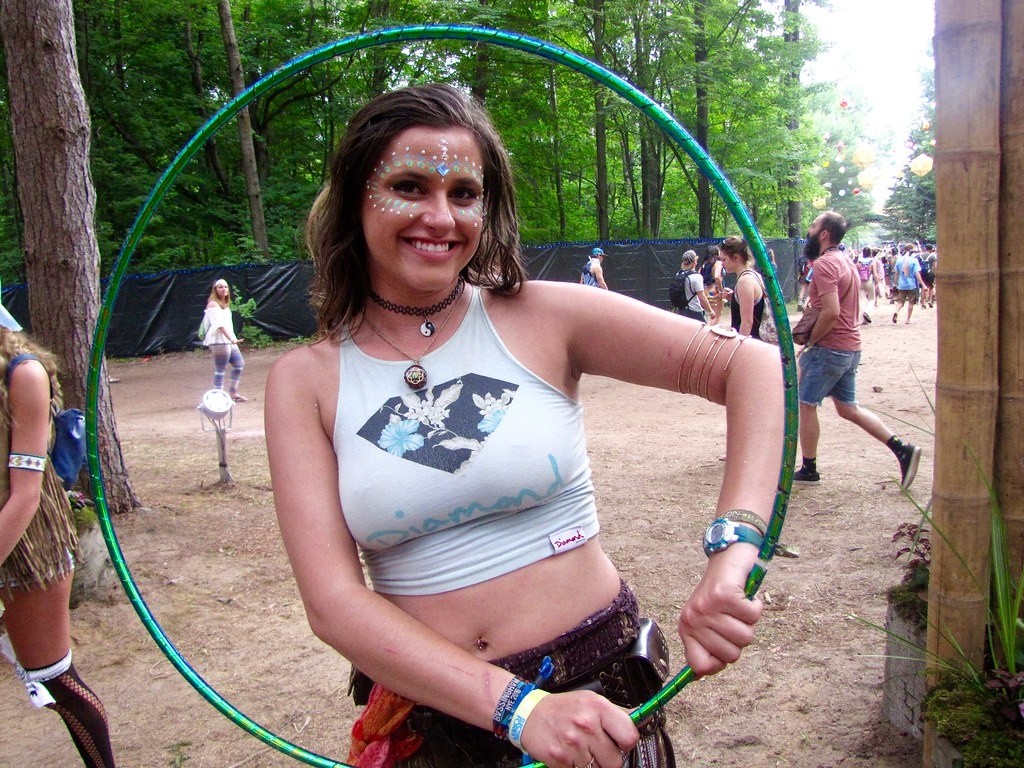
[368,277,462,337]
[359,279,466,388]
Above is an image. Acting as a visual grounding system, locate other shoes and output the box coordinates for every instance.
[906,321,912,325]
[892,312,898,323]
[791,470,821,486]
[921,305,927,309]
[797,300,804,311]
[862,312,872,324]
[901,442,922,491]
[890,300,894,304]
[928,301,933,308]
[230,395,248,402]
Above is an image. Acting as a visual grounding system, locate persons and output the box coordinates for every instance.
[719,237,766,341]
[792,211,922,491]
[676,250,716,325]
[855,247,879,323]
[698,244,726,326]
[756,249,779,342]
[264,84,800,768]
[796,242,937,314]
[892,244,927,324]
[580,248,608,290]
[202,278,247,401]
[0,303,115,767]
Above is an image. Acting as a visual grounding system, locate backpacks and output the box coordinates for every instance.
[700,255,723,284]
[797,255,812,272]
[857,258,874,281]
[917,253,933,274]
[734,263,781,346]
[197,300,221,340]
[669,269,704,310]
[5,351,86,491]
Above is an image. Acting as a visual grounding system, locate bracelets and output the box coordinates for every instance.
[492,656,554,740]
[715,509,768,535]
[509,689,551,755]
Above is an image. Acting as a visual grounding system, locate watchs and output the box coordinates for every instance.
[703,518,764,558]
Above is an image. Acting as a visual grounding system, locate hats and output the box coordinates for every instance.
[591,247,607,256]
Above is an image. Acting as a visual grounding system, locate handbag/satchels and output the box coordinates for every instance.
[791,307,820,345]
